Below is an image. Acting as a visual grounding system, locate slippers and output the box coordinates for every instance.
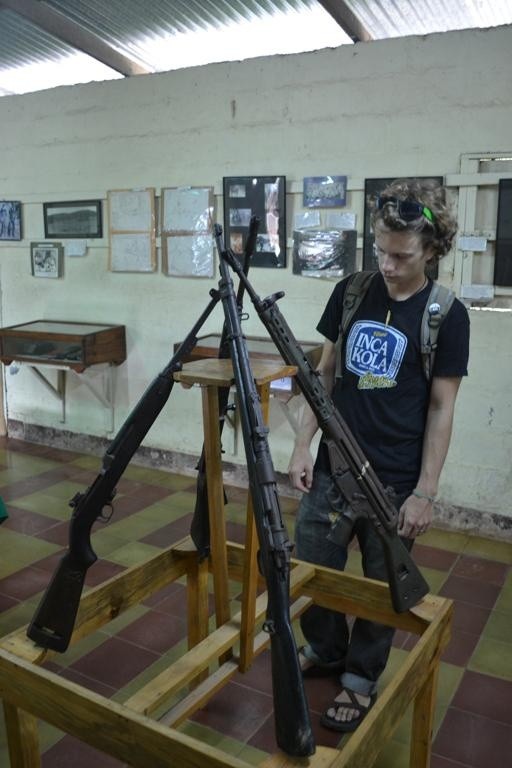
[319,688,376,731]
[296,644,346,677]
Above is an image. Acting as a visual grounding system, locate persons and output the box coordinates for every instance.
[287,175,470,731]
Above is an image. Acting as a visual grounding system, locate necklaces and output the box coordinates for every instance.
[379,277,426,327]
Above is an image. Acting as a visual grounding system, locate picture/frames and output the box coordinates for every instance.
[222,174,287,269]
[362,175,444,281]
[42,199,105,240]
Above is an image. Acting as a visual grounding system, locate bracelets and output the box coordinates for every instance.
[411,488,436,503]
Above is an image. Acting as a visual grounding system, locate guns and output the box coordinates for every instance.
[27,217,430,758]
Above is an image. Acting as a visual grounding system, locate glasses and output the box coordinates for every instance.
[374,193,432,222]
